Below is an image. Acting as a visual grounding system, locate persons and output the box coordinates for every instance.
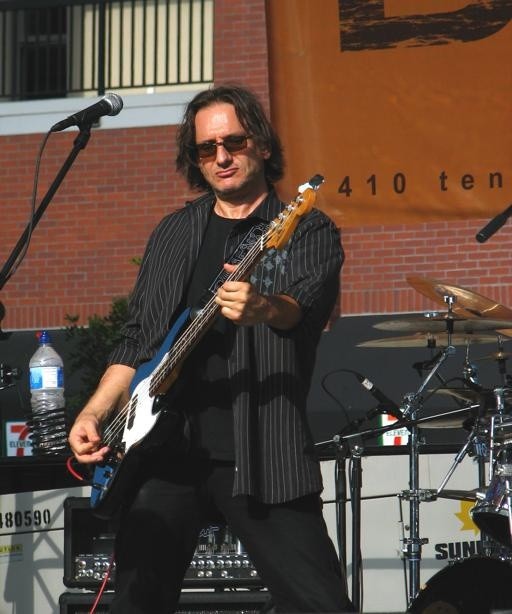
[68,82,355,613]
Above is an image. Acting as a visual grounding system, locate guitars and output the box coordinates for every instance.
[91,189,317,520]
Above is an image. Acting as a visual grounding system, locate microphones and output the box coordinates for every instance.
[50,93,124,132]
[358,375,425,446]
[474,203,512,244]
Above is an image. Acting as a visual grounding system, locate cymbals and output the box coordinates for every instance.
[428,387,512,410]
[355,274,511,348]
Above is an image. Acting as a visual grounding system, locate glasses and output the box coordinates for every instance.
[193,136,252,157]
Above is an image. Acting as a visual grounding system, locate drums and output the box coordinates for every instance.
[471,448,512,550]
[406,551,512,614]
[463,413,512,462]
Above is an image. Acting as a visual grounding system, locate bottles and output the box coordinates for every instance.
[27,331,69,456]
